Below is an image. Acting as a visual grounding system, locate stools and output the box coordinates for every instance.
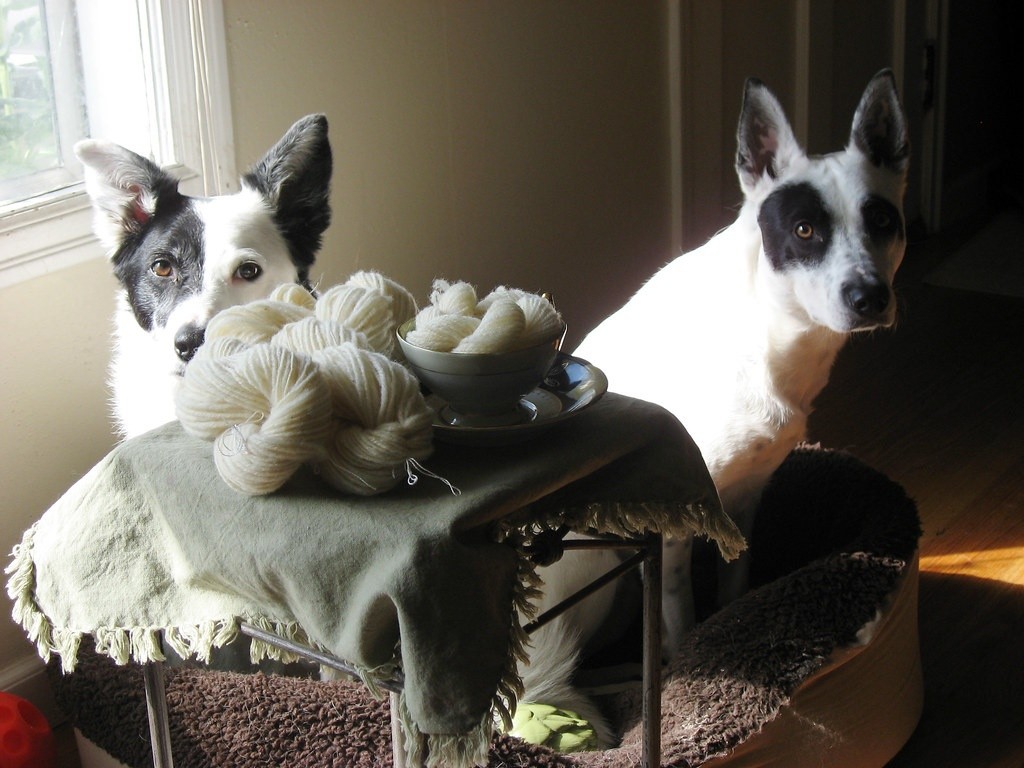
[112,391,667,767]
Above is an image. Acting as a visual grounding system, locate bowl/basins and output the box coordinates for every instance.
[397,312,567,428]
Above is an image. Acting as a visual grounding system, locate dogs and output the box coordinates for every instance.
[513,66,910,751]
[74,111,334,444]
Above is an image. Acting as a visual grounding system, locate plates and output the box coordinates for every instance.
[420,352,608,447]
